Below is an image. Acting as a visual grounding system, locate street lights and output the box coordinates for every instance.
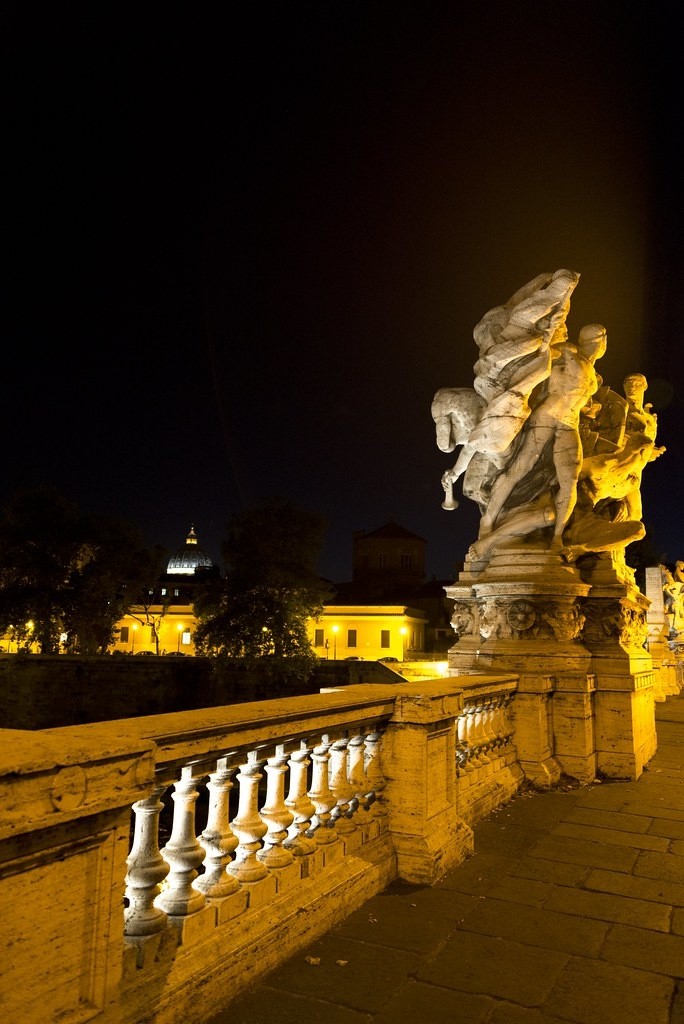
[132,623,137,655]
[332,624,338,660]
[176,623,183,657]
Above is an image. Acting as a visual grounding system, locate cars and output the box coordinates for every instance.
[135,651,154,656]
[344,656,364,662]
[377,657,399,663]
[166,652,183,656]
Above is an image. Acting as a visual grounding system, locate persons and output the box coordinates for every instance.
[658,560,684,631]
[442,270,666,564]
